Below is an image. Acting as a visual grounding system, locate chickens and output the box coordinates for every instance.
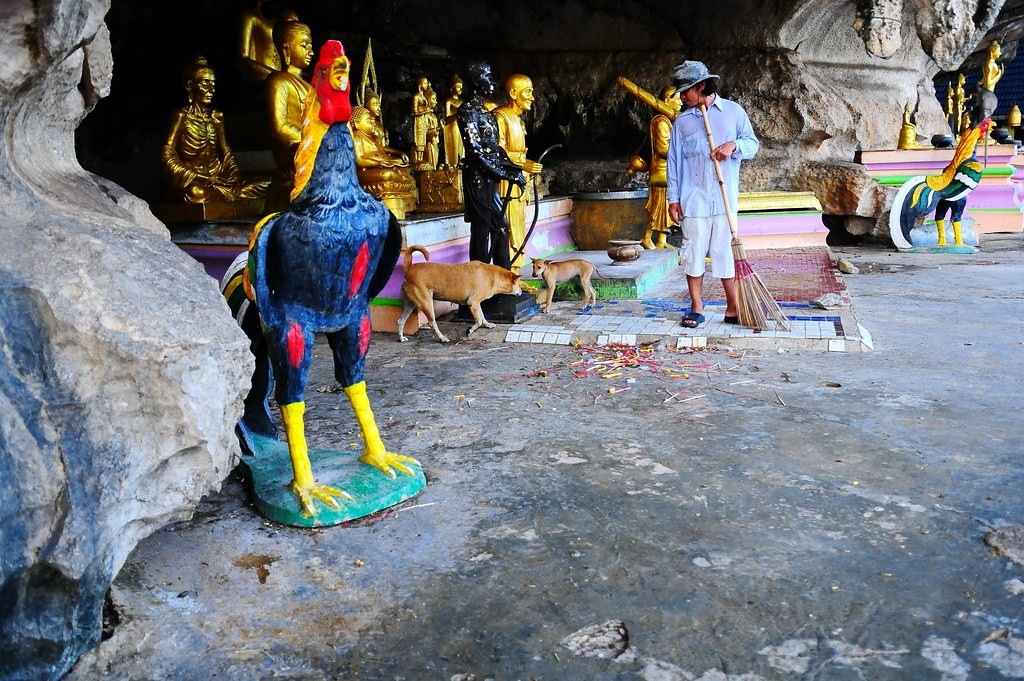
[219,39,421,520]
[888,115,997,250]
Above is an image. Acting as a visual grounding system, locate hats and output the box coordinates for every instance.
[670,60,721,98]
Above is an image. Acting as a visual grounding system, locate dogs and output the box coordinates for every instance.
[397,245,523,344]
[530,256,614,313]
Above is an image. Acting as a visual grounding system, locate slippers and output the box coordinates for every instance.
[724,313,747,325]
[680,312,705,327]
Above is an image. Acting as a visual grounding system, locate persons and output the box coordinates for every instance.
[161,56,273,206]
[963,111,971,130]
[350,89,411,182]
[982,41,1004,92]
[455,63,527,272]
[240,9,309,81]
[902,101,917,129]
[640,84,684,250]
[491,73,543,276]
[945,87,954,121]
[666,60,760,329]
[412,76,442,170]
[264,21,314,152]
[443,73,466,168]
[955,73,970,134]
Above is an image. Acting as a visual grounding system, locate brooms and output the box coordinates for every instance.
[701,104,795,333]
